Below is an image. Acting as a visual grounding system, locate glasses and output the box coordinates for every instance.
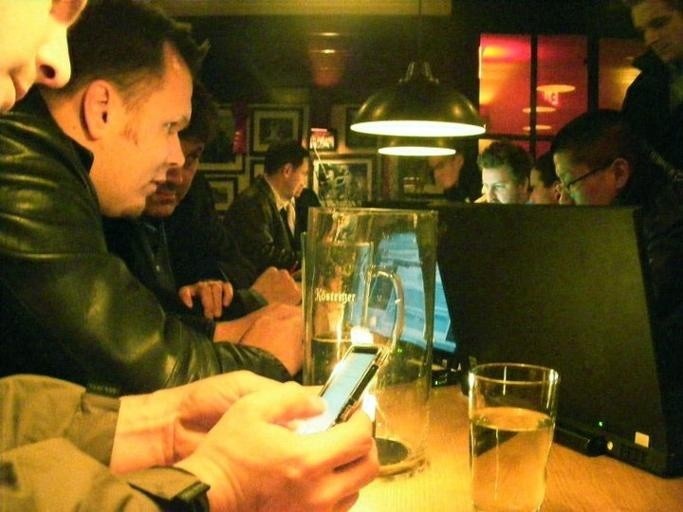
[555,163,608,196]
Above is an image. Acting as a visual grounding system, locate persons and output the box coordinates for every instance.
[0,0,380,512]
[626,2,683,167]
[0,0,307,393]
[280,188,322,256]
[104,84,301,320]
[530,154,563,204]
[222,142,307,289]
[474,141,532,206]
[426,143,470,201]
[555,109,682,310]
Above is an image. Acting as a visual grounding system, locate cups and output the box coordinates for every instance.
[303,205,438,478]
[464,361,561,511]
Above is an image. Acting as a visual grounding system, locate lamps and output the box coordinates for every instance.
[349,0,488,138]
[376,135,459,158]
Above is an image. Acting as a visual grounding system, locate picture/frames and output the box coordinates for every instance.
[252,160,308,200]
[330,103,384,155]
[309,127,337,151]
[383,155,443,203]
[210,180,236,214]
[196,102,244,175]
[310,154,378,207]
[250,106,303,155]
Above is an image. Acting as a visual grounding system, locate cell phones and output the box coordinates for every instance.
[296,345,389,433]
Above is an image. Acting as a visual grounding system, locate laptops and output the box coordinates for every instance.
[372,225,459,388]
[427,201,683,479]
